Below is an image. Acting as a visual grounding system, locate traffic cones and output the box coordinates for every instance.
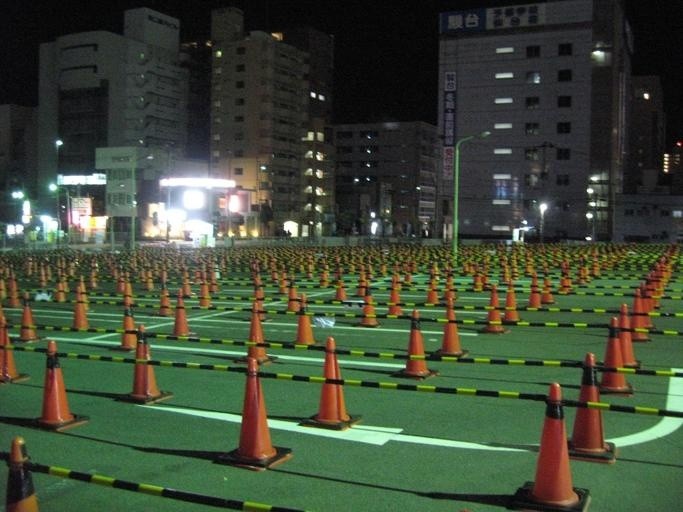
[34,339,92,432]
[291,293,316,349]
[598,316,635,395]
[433,302,470,359]
[216,357,294,470]
[114,325,176,406]
[4,434,41,512]
[299,334,362,432]
[506,380,592,512]
[0,312,32,385]
[120,302,139,350]
[617,303,642,371]
[393,309,441,380]
[236,310,279,366]
[566,350,619,466]
[0,243,682,339]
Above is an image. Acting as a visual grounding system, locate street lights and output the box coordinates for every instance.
[454,130,491,260]
